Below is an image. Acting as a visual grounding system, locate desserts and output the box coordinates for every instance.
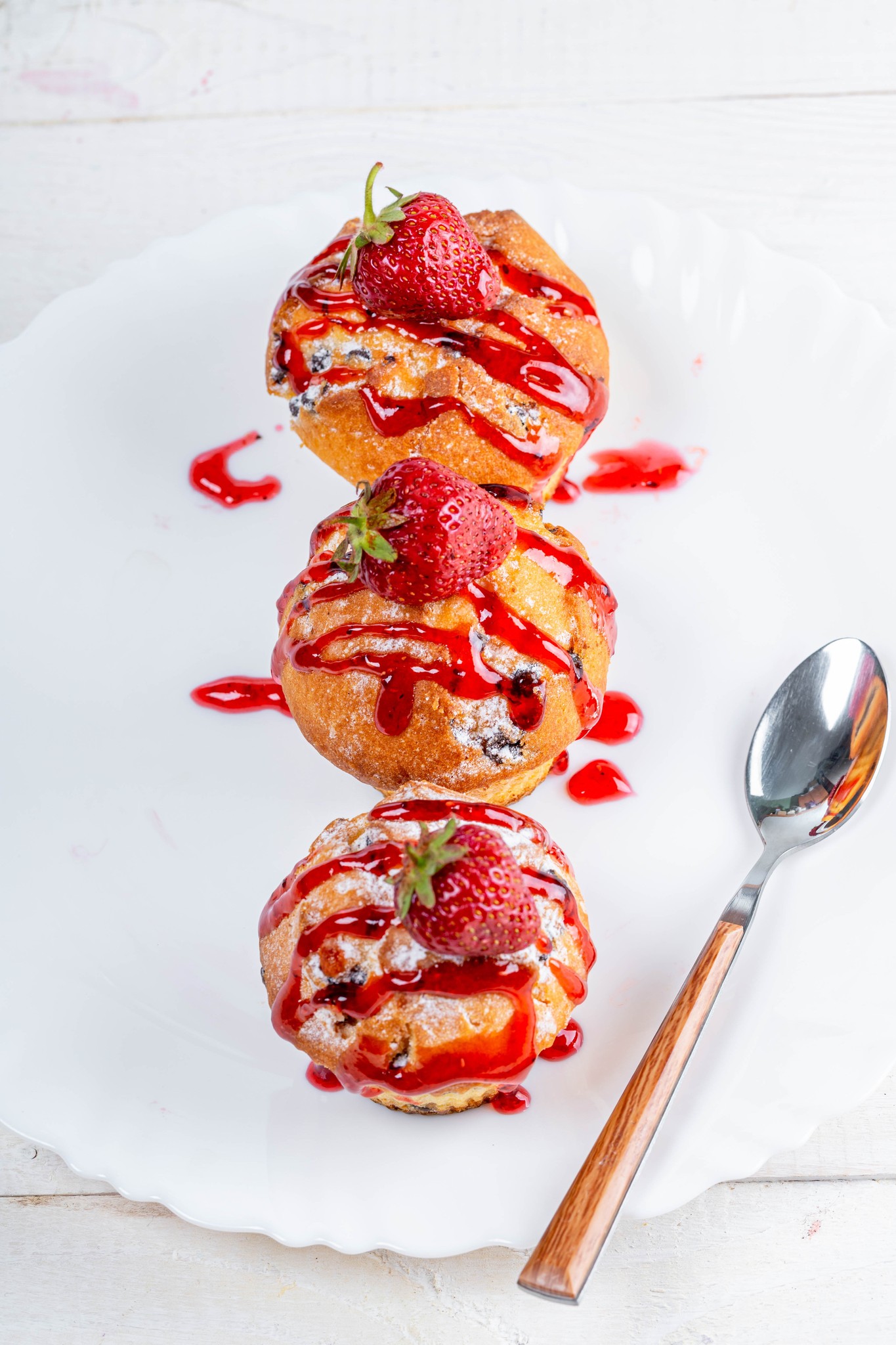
[263,163,609,514]
[257,778,595,1113]
[270,458,621,809]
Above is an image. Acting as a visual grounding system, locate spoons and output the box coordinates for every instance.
[518,630,892,1305]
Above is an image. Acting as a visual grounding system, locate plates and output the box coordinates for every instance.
[0,195,896,1251]
